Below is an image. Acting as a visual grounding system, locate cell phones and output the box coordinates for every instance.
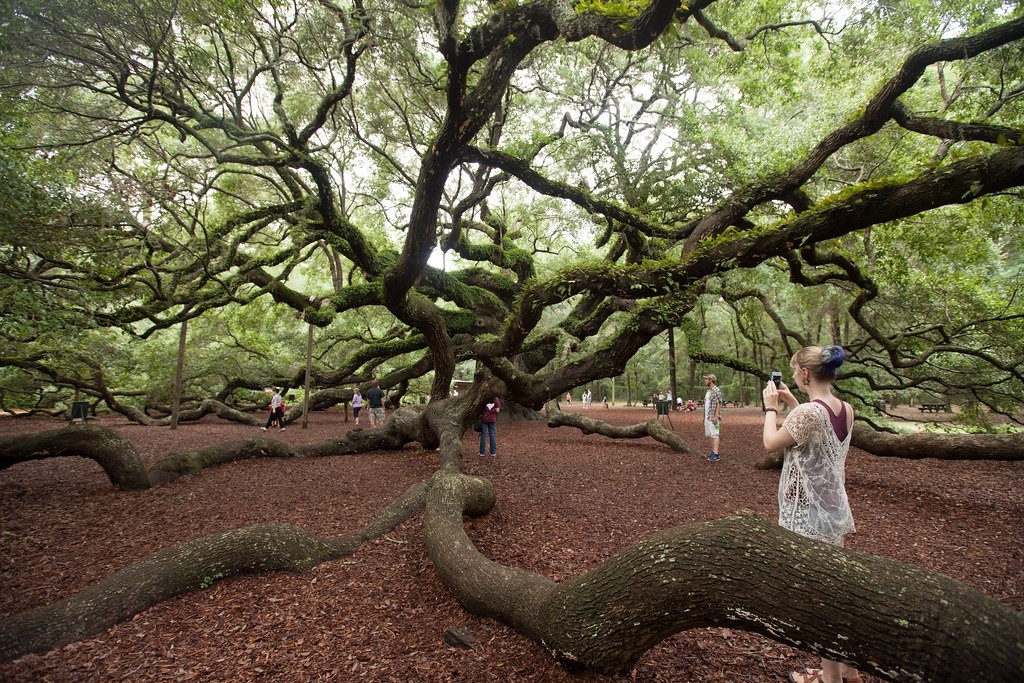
[771,372,782,390]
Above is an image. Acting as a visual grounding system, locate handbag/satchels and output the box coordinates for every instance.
[474,418,483,432]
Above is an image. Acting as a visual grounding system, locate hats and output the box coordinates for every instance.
[703,374,716,381]
[353,388,359,391]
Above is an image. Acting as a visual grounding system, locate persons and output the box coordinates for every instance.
[427,394,431,401]
[477,397,501,457]
[659,392,666,401]
[602,396,608,409]
[453,390,458,396]
[762,344,866,683]
[666,389,672,409]
[566,393,571,406]
[419,395,425,404]
[677,396,695,412]
[351,388,363,425]
[384,397,394,409]
[260,387,286,432]
[367,380,386,428]
[703,374,722,461]
[582,389,592,409]
[652,394,658,411]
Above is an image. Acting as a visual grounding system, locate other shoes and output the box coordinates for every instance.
[491,454,496,456]
[704,451,720,461]
[279,428,285,432]
[260,427,267,431]
[477,453,485,457]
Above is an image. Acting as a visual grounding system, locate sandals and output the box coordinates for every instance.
[789,668,866,683]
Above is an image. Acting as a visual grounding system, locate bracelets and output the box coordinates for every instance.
[764,408,777,415]
